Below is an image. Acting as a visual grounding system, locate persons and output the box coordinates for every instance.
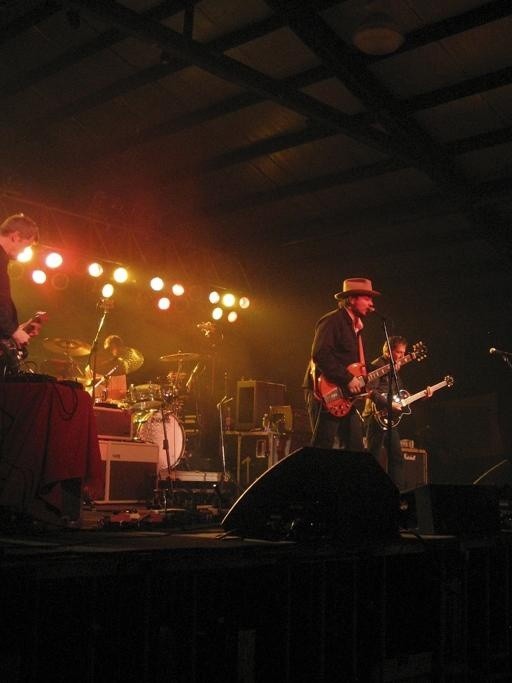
[362,335,434,492]
[85,332,129,395]
[0,211,42,371]
[301,276,406,449]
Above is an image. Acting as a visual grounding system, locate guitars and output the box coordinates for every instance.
[372,376,454,430]
[0,311,47,366]
[317,342,427,417]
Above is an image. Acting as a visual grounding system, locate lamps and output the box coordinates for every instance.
[353,12,407,58]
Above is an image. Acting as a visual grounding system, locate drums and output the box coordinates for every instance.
[126,384,163,410]
[131,409,186,471]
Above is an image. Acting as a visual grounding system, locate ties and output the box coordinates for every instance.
[353,317,365,367]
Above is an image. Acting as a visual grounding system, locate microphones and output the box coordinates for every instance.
[489,347,512,357]
[185,364,200,386]
[368,306,389,322]
[197,323,215,332]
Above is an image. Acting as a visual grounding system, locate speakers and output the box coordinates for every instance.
[402,483,503,536]
[218,447,401,542]
[93,407,133,442]
[94,441,160,505]
[238,432,301,498]
[379,447,429,492]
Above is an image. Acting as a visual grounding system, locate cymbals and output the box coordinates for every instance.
[89,346,144,375]
[43,338,92,355]
[159,354,200,362]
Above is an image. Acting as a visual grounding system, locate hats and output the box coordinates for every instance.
[334,277,380,302]
